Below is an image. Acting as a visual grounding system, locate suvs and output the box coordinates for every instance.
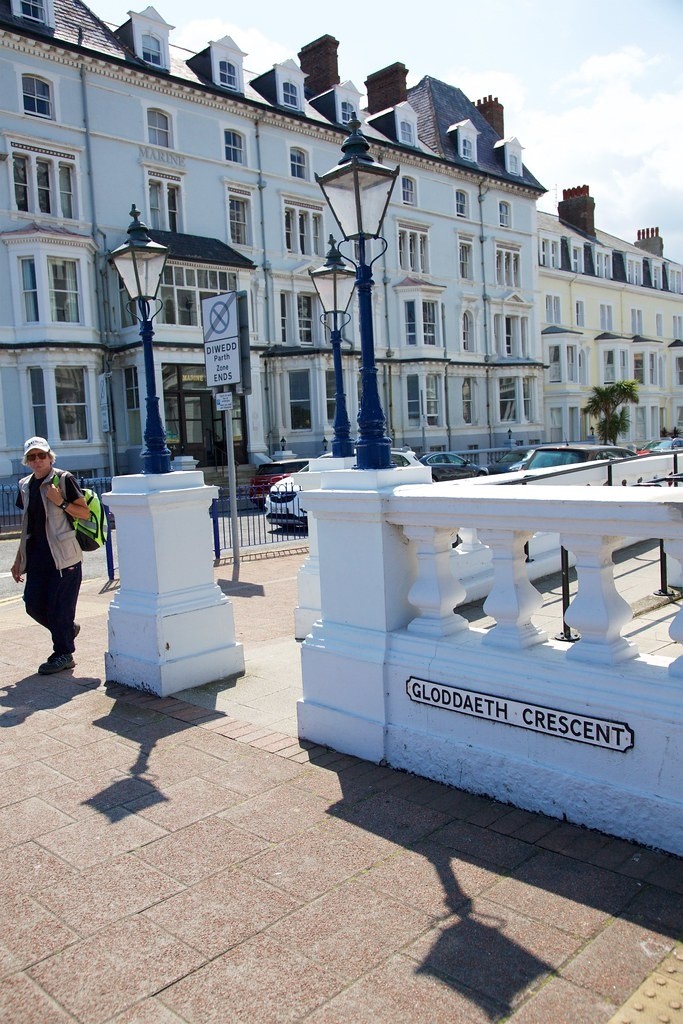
[247,457,312,510]
[263,446,426,529]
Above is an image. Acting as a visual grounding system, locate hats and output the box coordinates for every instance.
[24,437,50,455]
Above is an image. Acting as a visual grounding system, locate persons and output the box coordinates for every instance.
[10,437,90,674]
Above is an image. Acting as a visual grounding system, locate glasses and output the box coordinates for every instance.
[27,452,47,461]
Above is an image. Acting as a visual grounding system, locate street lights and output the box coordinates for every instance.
[310,233,358,467]
[314,110,403,469]
[103,204,175,476]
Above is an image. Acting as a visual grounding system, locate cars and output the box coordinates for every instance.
[521,443,638,475]
[488,443,546,476]
[416,453,489,485]
[638,438,683,455]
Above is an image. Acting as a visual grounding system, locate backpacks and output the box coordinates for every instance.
[53,471,108,551]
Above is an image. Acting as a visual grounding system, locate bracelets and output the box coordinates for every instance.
[58,499,69,510]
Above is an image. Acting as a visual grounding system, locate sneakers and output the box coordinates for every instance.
[73,622,80,638]
[38,651,75,673]
